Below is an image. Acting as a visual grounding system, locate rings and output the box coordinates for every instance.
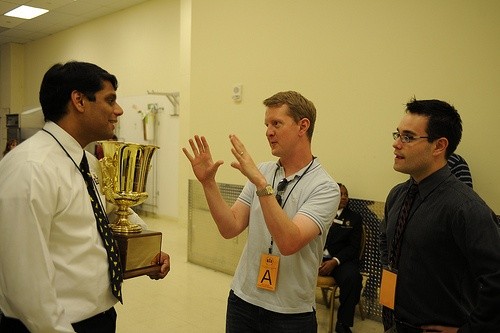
[240,152,243,156]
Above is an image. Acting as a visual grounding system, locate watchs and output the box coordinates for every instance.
[256,184,273,196]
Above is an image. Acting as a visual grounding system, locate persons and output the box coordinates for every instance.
[183,90,341,333]
[319,183,364,333]
[381,95,500,333]
[447,153,473,189]
[0,61,170,333]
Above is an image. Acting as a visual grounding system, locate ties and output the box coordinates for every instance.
[80,150,123,304]
[382,184,416,333]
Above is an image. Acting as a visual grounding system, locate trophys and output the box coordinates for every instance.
[96,139,162,279]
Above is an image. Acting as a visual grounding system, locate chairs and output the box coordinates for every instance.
[317,224,366,333]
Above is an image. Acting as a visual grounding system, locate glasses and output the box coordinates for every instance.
[393,132,442,143]
